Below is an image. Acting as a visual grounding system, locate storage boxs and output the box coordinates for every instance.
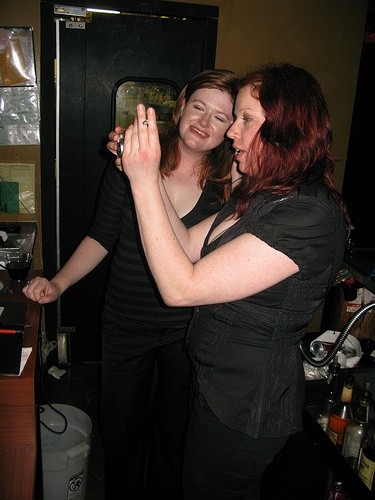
[320,282,375,339]
[302,378,375,500]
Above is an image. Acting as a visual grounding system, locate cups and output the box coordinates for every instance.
[3,252,35,284]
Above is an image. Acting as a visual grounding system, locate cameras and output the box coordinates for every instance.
[117,135,124,156]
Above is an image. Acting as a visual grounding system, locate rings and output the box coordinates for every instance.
[27,282,30,286]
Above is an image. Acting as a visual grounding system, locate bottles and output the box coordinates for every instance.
[324,374,356,448]
[341,383,373,473]
[315,362,344,434]
[358,425,375,496]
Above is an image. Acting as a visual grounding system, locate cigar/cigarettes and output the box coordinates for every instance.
[143,120,148,125]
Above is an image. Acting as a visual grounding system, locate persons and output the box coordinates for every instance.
[107,62,349,500]
[22,69,240,500]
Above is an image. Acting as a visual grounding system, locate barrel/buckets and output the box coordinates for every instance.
[36,403,93,500]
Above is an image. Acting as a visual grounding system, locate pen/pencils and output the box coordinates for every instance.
[143,119,166,124]
[0,329,20,334]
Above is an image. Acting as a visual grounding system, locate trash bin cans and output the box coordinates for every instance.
[40,403,93,500]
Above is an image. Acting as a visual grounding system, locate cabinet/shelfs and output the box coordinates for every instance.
[0,267,44,500]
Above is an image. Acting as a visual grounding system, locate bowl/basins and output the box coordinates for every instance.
[0,224,36,261]
